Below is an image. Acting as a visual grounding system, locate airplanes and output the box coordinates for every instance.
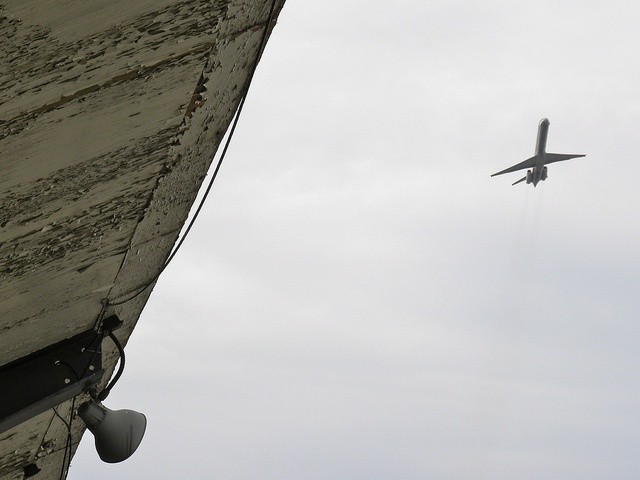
[491,119,586,188]
